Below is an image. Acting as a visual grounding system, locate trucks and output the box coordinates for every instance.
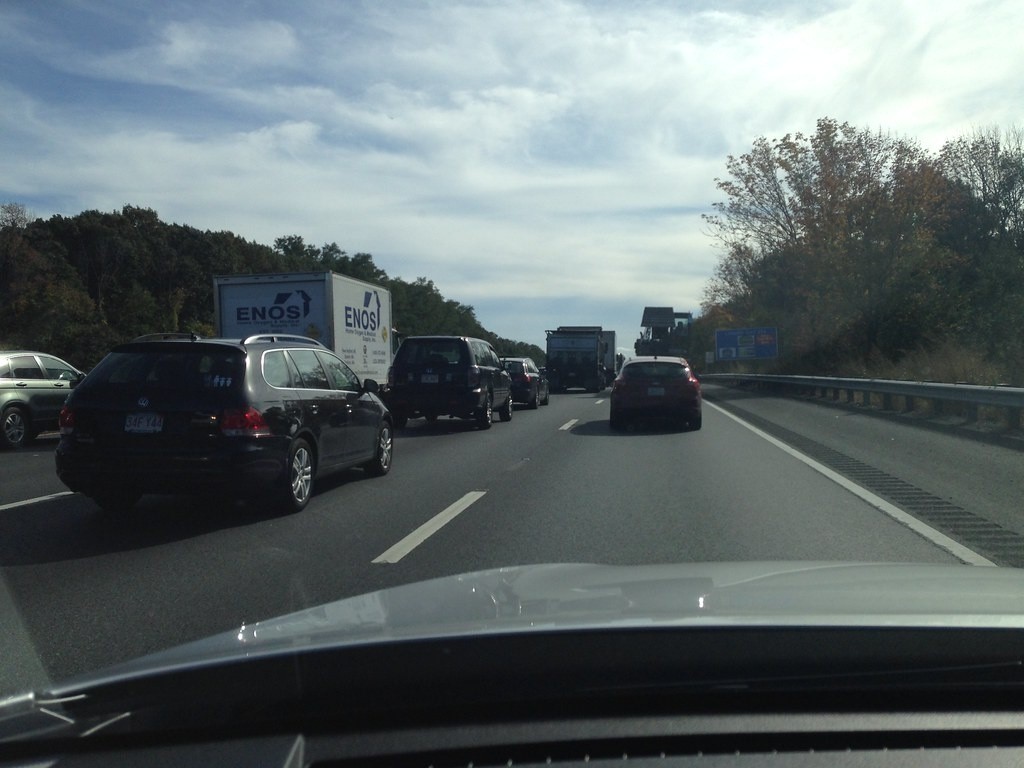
[214,270,401,389]
[544,326,608,392]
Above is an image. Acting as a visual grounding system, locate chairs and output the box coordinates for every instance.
[629,365,645,376]
[425,354,449,365]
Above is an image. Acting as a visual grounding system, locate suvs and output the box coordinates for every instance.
[55,332,393,514]
[386,336,512,428]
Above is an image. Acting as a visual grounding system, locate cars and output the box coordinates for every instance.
[0,350,88,448]
[610,356,702,429]
[500,357,548,410]
